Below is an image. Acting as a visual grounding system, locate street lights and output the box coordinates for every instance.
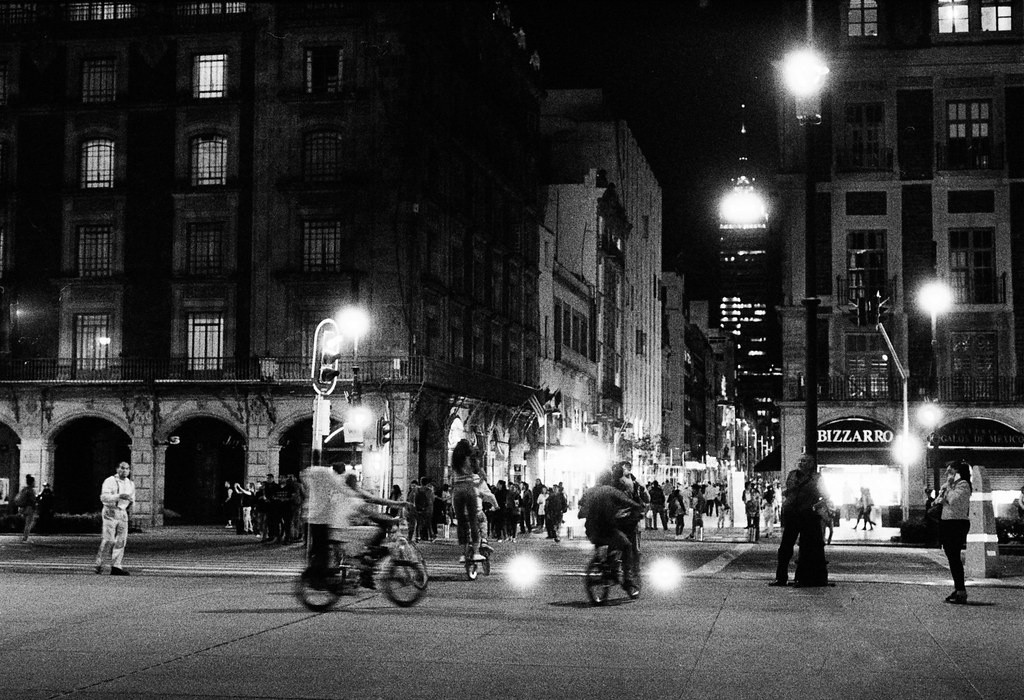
[775,42,834,586]
[912,279,953,498]
[335,307,370,470]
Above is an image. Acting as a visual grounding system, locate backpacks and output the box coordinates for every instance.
[15,488,30,506]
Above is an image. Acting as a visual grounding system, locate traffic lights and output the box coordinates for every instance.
[310,317,343,396]
[376,415,392,447]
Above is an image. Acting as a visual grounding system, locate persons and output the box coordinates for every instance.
[579,461,650,595]
[89,462,137,576]
[0,474,55,543]
[1018,485,1024,523]
[645,473,787,544]
[852,486,877,531]
[769,455,834,587]
[931,462,973,604]
[223,438,570,582]
[925,488,935,513]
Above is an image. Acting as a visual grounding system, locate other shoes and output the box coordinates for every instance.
[22,539,34,543]
[480,542,494,552]
[459,556,466,562]
[94,566,103,574]
[111,566,129,575]
[770,579,786,586]
[945,592,968,603]
[472,554,486,561]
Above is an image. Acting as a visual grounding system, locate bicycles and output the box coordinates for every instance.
[459,506,502,582]
[584,499,651,607]
[291,502,428,612]
